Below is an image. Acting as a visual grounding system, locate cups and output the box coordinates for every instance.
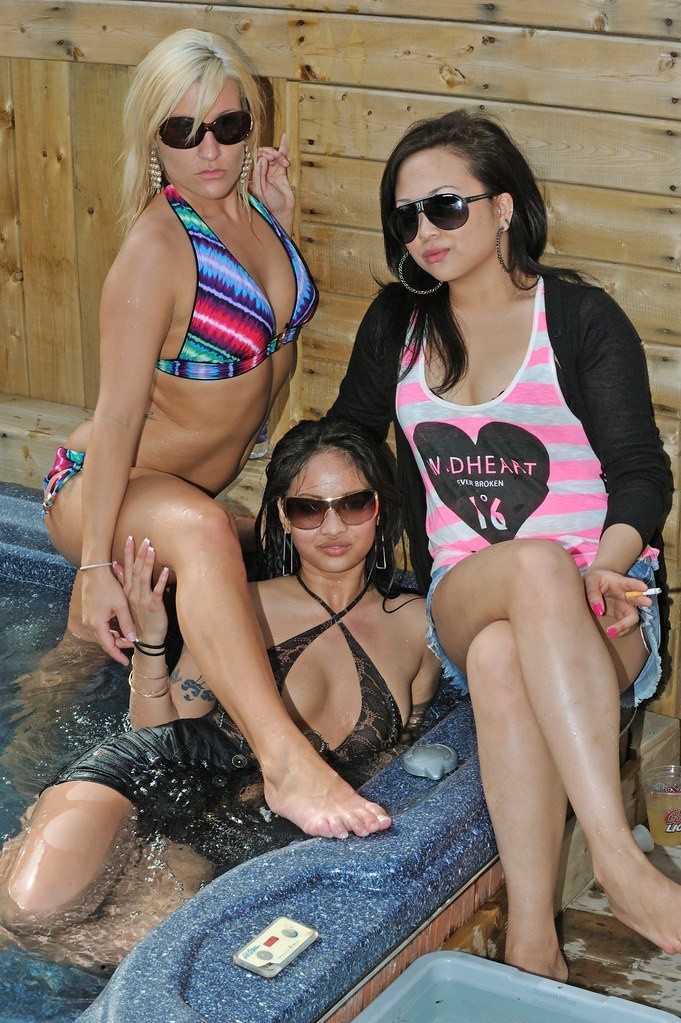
[631,824,654,853]
[641,765,681,846]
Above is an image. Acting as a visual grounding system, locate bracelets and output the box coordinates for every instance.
[131,655,171,680]
[80,563,113,570]
[127,671,171,698]
[133,641,167,658]
[138,640,165,649]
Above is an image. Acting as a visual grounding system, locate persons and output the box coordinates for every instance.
[42,24,394,841]
[329,107,680,980]
[7,418,440,920]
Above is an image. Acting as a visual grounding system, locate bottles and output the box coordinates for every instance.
[249,420,269,460]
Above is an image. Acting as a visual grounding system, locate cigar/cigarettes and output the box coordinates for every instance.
[626,587,663,599]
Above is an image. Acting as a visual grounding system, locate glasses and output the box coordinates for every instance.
[158,110,253,150]
[281,487,379,530]
[386,191,498,244]
